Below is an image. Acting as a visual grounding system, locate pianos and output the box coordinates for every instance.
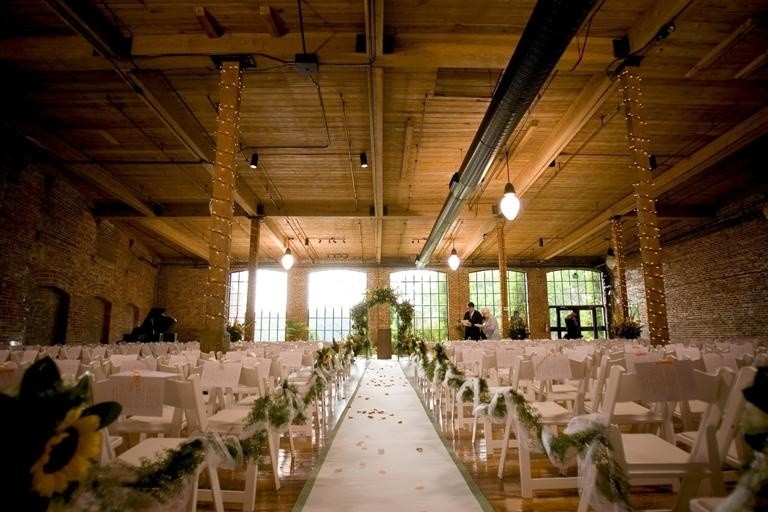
[124,307,177,342]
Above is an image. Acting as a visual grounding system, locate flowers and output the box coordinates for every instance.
[347,283,417,356]
[504,316,527,332]
[606,300,644,339]
[225,315,256,342]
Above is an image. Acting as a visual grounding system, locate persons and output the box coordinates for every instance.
[476,308,501,339]
[508,311,526,340]
[463,303,483,340]
[565,312,580,339]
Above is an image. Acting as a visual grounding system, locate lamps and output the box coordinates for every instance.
[358,153,369,168]
[648,153,656,172]
[497,150,522,223]
[445,238,464,274]
[247,152,258,169]
[303,237,310,248]
[279,236,299,272]
[604,235,618,272]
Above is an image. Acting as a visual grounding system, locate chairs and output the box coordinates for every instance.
[0,334,356,512]
[408,331,767,512]
